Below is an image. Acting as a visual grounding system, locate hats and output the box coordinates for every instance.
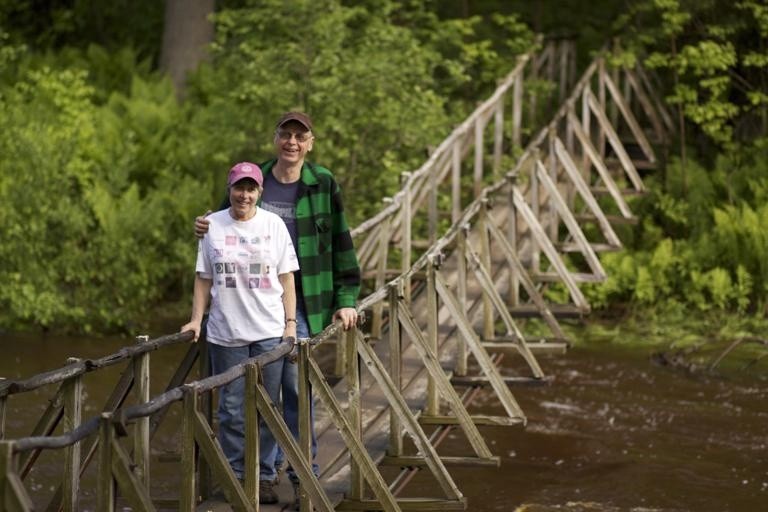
[277,111,313,131]
[227,162,263,190]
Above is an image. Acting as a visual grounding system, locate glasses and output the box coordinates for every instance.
[278,132,312,142]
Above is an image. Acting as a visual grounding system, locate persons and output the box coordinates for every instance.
[181,162,300,507]
[194,110,363,509]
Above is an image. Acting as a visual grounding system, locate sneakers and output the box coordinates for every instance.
[224,478,245,503]
[293,482,315,512]
[273,459,290,485]
[259,479,279,504]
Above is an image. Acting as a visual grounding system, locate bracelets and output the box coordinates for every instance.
[285,318,298,324]
